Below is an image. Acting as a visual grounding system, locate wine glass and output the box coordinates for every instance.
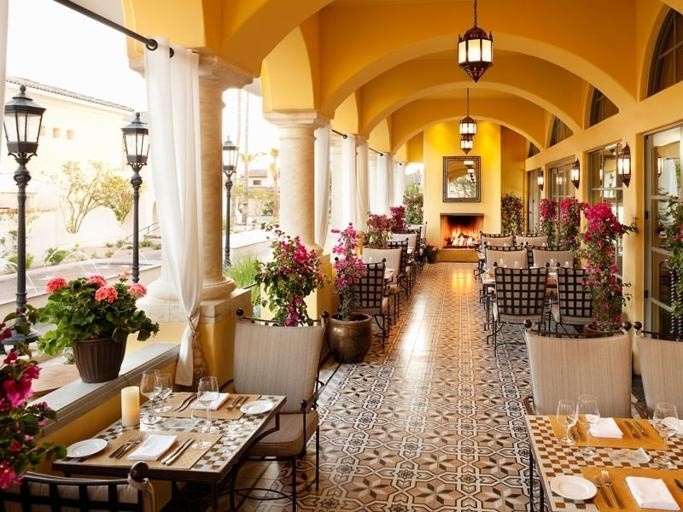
[196,377,219,434]
[480,241,547,257]
[652,404,678,470]
[481,258,570,278]
[153,374,172,413]
[575,393,599,457]
[140,369,161,425]
[556,399,575,446]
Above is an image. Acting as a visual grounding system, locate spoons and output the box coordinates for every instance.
[236,396,248,408]
[108,433,138,459]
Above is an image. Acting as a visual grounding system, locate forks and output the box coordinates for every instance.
[600,471,623,510]
[114,434,144,460]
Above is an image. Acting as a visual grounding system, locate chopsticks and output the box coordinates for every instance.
[172,393,196,412]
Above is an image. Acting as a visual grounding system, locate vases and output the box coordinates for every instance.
[427,255,435,263]
[70,334,128,381]
[584,322,622,338]
[328,313,372,362]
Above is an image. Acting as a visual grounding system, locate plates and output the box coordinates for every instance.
[549,476,596,500]
[66,439,107,458]
[239,400,273,415]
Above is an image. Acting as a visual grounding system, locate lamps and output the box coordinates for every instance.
[459,135,472,154]
[459,88,477,138]
[537,170,543,190]
[617,145,630,186]
[457,0,492,81]
[570,161,579,189]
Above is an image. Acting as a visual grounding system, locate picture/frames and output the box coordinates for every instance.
[443,155,481,203]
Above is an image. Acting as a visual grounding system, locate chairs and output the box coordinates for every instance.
[634,322,683,417]
[521,319,633,511]
[515,233,548,248]
[333,257,390,350]
[552,262,595,337]
[491,261,550,357]
[217,308,326,511]
[530,244,574,268]
[361,247,400,324]
[408,221,426,248]
[387,237,411,297]
[483,247,528,272]
[391,233,417,283]
[19,463,154,512]
[404,227,422,274]
[474,230,513,280]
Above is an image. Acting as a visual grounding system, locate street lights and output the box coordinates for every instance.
[3,85,46,154]
[221,136,239,174]
[121,112,150,165]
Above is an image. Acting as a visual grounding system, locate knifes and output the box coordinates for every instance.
[160,437,195,465]
[593,476,612,508]
[225,396,243,409]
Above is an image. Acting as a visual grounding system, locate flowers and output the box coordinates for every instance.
[425,242,438,258]
[35,266,159,358]
[328,223,373,319]
